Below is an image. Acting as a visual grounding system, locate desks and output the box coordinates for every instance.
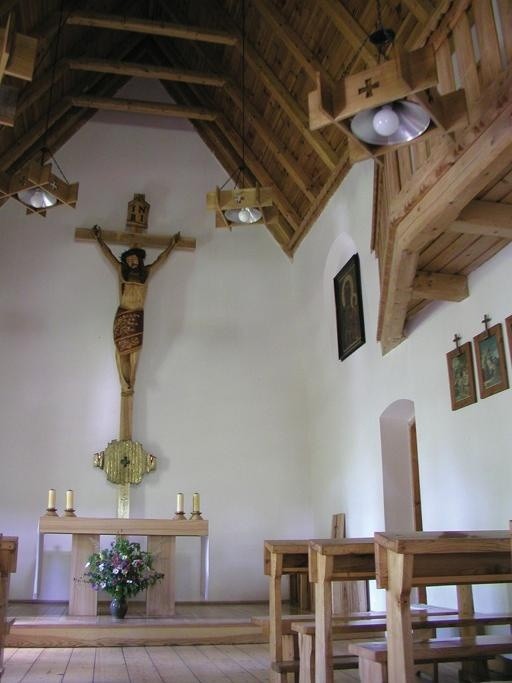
[0,531,19,682]
[30,514,212,622]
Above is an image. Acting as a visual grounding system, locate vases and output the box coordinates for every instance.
[110,596,129,620]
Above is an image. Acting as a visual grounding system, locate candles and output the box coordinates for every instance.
[176,491,185,513]
[47,487,58,510]
[66,488,75,509]
[193,490,200,513]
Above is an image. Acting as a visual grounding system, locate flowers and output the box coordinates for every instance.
[73,530,166,599]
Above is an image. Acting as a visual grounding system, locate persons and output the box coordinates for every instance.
[91,225,181,394]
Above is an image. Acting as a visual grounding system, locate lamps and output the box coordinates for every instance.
[304,0,475,165]
[0,0,82,219]
[205,1,280,229]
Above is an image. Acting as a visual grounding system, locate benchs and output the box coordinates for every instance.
[251,527,512,682]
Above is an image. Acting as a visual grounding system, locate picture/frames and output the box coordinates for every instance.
[445,340,478,412]
[333,251,367,362]
[472,322,511,399]
